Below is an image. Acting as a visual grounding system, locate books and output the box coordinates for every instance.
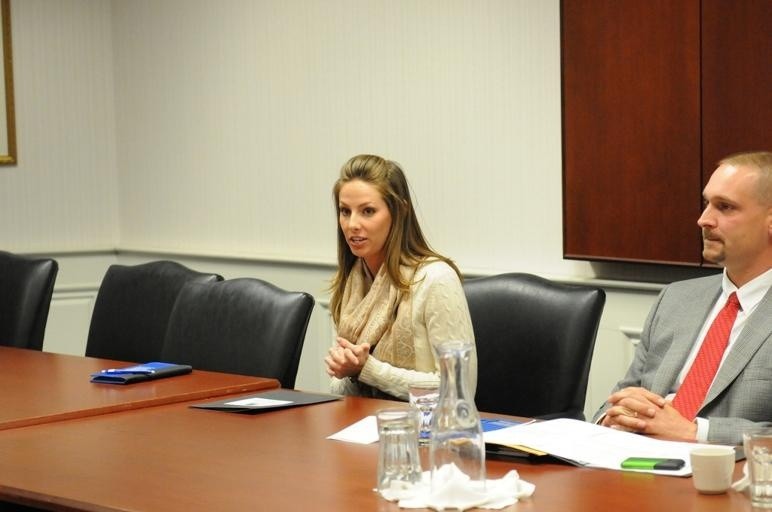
[449,435,549,460]
[411,408,535,444]
[89,362,193,385]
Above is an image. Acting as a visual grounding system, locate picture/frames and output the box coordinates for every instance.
[0,0,19,166]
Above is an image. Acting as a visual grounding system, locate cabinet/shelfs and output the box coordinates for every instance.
[561,0,772,270]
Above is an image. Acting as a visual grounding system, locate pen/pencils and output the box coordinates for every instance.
[100,369,154,374]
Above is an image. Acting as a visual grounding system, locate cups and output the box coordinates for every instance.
[376,407,419,496]
[410,383,441,443]
[743,422,772,511]
[689,447,736,496]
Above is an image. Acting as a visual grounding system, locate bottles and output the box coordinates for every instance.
[428,342,486,481]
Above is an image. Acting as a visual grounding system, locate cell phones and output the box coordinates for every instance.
[622,457,685,470]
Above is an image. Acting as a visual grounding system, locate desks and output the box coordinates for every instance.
[0,343,282,441]
[0,384,772,512]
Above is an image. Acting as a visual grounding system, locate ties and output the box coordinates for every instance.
[669,291,742,421]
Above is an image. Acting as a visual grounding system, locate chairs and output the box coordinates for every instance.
[159,277,316,391]
[460,271,606,422]
[85,260,225,363]
[0,249,59,351]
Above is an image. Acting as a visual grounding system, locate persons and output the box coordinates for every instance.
[322,154,480,408]
[589,149,772,446]
